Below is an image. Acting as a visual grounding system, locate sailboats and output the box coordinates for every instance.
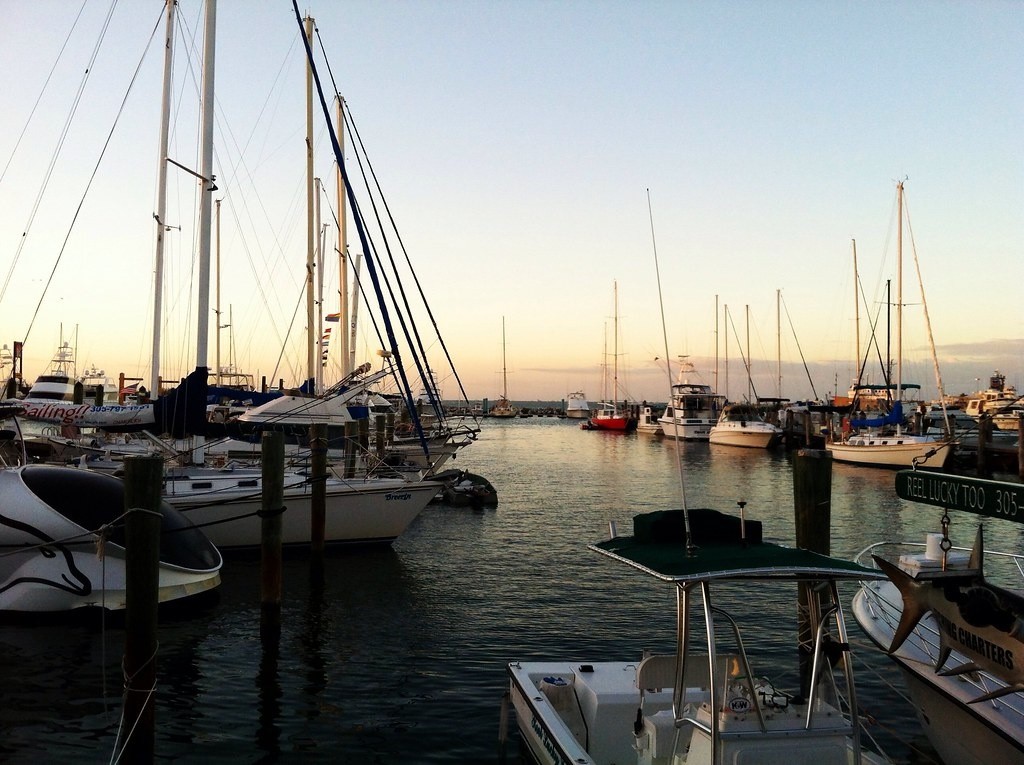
[485,316,520,417]
[0,0,495,618]
[590,175,1024,478]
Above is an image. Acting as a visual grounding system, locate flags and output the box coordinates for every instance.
[319,312,341,368]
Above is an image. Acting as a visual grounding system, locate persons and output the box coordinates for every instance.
[842,414,850,441]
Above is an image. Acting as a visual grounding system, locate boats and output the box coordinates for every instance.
[849,538,1024,752]
[502,187,988,765]
[565,392,590,419]
